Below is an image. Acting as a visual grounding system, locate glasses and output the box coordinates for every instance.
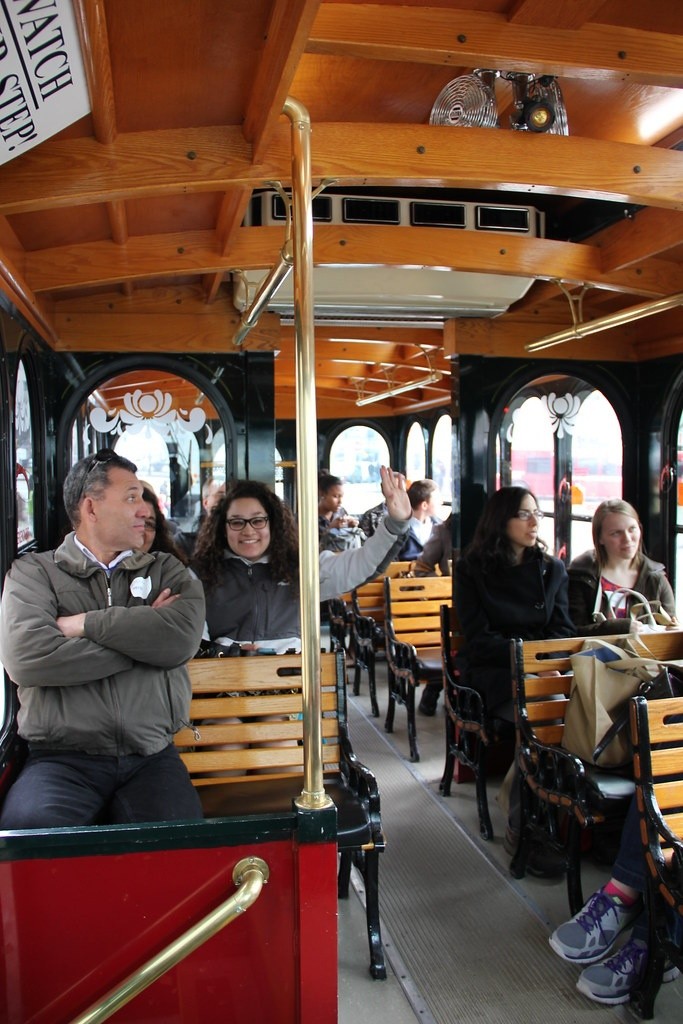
[225,516,269,531]
[76,448,118,504]
[509,510,545,521]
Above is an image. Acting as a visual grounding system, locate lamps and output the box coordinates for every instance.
[508,68,556,133]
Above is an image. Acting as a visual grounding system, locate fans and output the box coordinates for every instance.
[429,68,569,136]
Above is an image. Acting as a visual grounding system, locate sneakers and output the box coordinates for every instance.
[503,813,569,879]
[576,936,679,1004]
[548,884,635,964]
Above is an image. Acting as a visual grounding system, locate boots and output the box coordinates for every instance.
[420,680,443,715]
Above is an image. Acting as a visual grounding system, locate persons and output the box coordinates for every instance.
[567,499,682,637]
[453,487,617,877]
[0,448,206,829]
[549,713,683,1004]
[190,465,414,778]
[295,469,452,716]
[139,476,227,569]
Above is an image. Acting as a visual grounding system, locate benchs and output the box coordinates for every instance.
[174,562,683,1019]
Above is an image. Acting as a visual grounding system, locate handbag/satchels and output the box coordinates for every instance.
[320,526,368,553]
[398,560,433,591]
[559,639,683,772]
[191,638,327,702]
[608,586,673,633]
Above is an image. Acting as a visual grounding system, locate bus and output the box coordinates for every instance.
[677,451,683,506]
[495,449,554,497]
[572,456,621,500]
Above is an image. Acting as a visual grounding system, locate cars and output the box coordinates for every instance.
[414,458,445,489]
[160,472,225,515]
